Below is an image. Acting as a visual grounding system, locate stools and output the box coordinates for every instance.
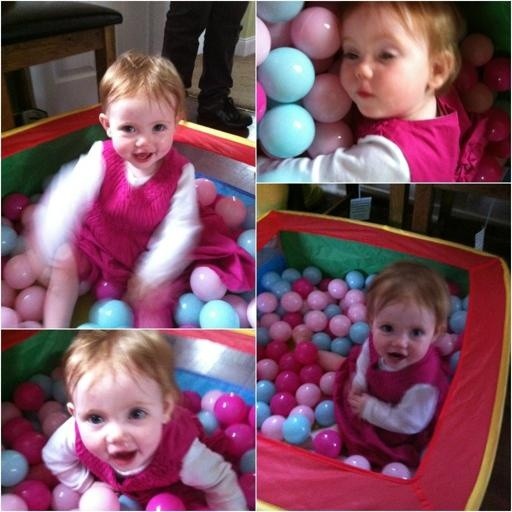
[2,2,123,132]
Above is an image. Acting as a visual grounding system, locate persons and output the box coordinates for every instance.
[1,177,257,328]
[31,49,197,329]
[256,2,510,182]
[161,1,253,129]
[41,328,249,510]
[258,264,469,480]
[1,367,255,511]
